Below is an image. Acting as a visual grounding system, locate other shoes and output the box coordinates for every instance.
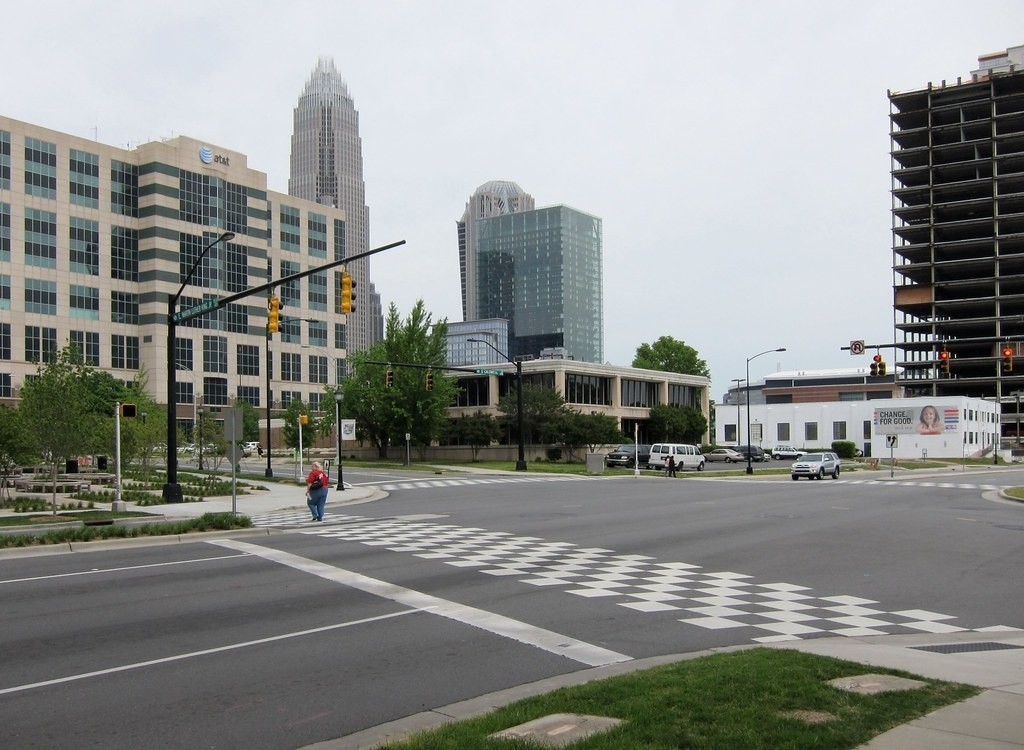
[313,516,322,521]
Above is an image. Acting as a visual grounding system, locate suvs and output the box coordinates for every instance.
[734,446,764,462]
[771,444,807,460]
[791,451,841,480]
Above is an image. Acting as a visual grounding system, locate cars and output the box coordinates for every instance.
[702,448,745,464]
[153,442,217,453]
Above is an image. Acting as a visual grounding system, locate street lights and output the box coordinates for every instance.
[198,404,204,470]
[264,318,319,479]
[141,412,146,465]
[332,386,345,491]
[731,346,789,475]
[165,230,235,484]
[301,344,340,466]
[468,338,528,471]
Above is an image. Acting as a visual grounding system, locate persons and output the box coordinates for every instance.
[256,443,263,462]
[305,462,328,521]
[664,456,677,477]
[915,405,944,435]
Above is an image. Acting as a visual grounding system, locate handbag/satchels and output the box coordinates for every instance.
[309,471,323,490]
[261,450,263,453]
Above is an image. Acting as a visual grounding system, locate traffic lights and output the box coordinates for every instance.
[870,354,886,376]
[301,416,309,424]
[940,351,949,373]
[1003,349,1012,371]
[268,298,283,332]
[386,371,393,387]
[426,374,433,391]
[341,274,357,314]
[120,404,138,418]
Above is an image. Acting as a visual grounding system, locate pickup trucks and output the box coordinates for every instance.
[604,444,652,468]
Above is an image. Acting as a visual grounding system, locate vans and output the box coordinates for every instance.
[647,443,705,471]
[244,441,260,453]
